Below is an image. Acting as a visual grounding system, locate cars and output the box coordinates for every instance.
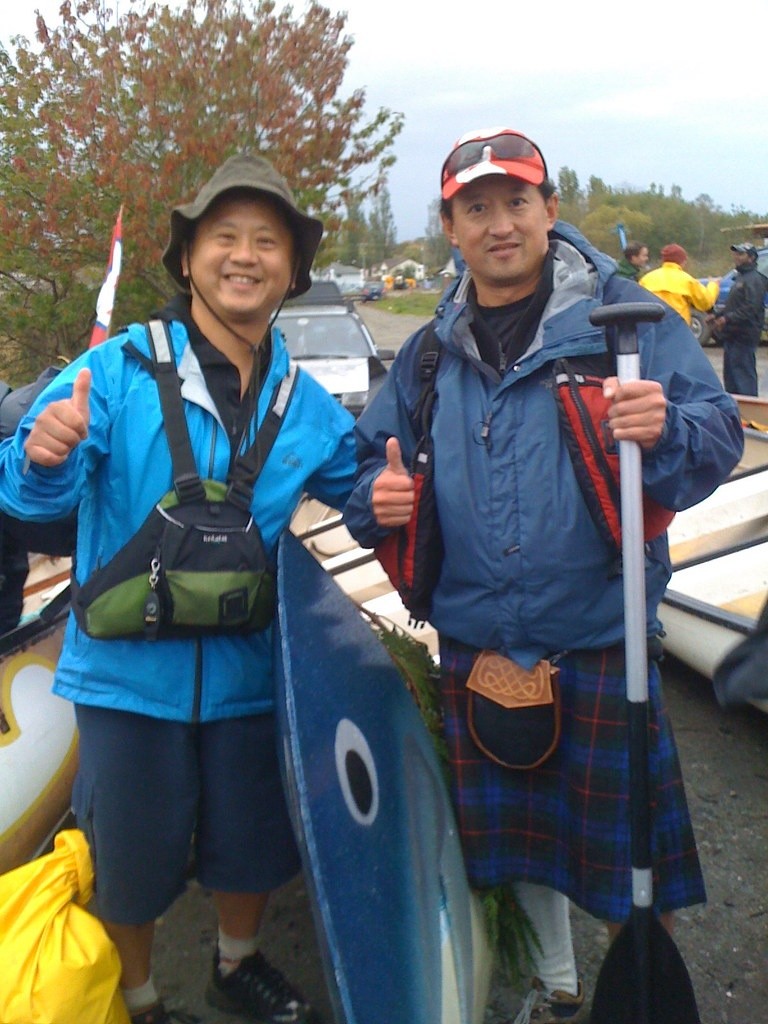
[276,280,397,418]
[362,280,386,302]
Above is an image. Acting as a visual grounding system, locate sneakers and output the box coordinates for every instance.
[132,1000,174,1024]
[203,941,312,1024]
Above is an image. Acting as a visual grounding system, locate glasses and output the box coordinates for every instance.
[441,133,543,189]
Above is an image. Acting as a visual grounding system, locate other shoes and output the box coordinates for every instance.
[512,972,583,1024]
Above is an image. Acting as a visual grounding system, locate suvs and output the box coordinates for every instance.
[685,247,768,352]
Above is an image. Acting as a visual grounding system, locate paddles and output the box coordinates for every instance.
[591,299,704,1024]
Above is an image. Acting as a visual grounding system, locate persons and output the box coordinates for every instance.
[706,243,765,397]
[637,244,722,327]
[0,154,362,1024]
[610,240,649,284]
[345,132,744,1024]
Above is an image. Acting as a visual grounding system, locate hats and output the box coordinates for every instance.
[660,245,686,263]
[162,154,325,300]
[439,130,548,201]
[730,241,758,257]
